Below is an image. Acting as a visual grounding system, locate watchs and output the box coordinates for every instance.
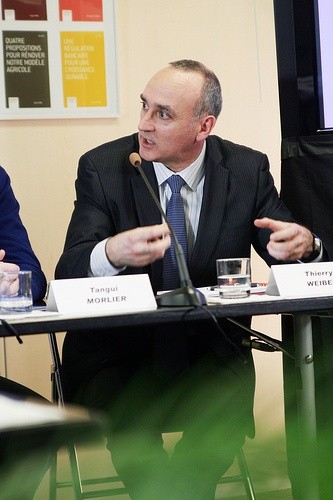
[300,233,321,263]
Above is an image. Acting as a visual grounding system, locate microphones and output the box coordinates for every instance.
[129,152,208,307]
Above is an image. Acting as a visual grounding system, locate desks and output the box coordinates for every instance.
[0,281,333,500]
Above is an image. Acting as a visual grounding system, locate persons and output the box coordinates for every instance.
[55,60,333,500]
[0,163,59,500]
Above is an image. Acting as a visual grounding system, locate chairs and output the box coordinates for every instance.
[48,330,264,500]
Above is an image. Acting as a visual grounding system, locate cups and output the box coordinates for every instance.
[216,258,252,299]
[0,270,33,319]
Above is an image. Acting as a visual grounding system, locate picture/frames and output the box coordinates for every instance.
[0,0,122,120]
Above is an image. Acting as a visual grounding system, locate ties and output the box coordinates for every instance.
[163,176,188,290]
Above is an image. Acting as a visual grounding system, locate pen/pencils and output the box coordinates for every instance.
[211,282,259,291]
